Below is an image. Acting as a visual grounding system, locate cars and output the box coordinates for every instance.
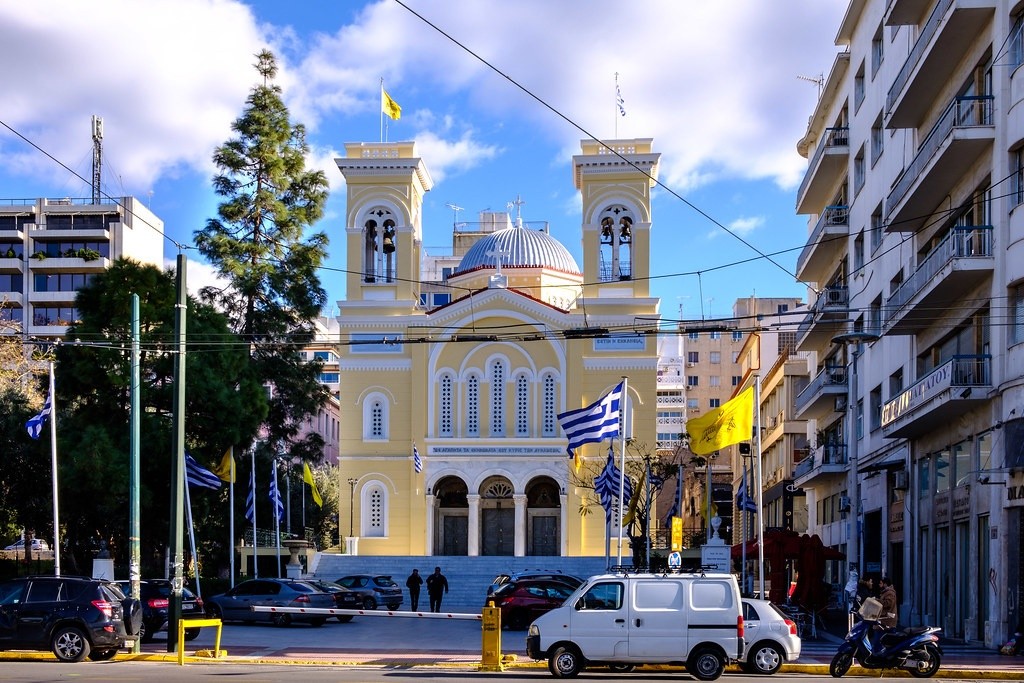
[739,598,802,675]
[204,577,364,628]
[5,539,48,551]
[484,578,580,631]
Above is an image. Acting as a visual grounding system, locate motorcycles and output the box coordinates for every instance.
[829,590,945,678]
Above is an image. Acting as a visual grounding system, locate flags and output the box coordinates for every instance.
[699,489,718,528]
[684,385,754,454]
[617,86,626,116]
[737,478,756,513]
[556,380,623,459]
[414,448,424,473]
[664,470,680,528]
[25,375,55,439]
[212,448,236,483]
[184,450,221,490]
[383,90,402,121]
[589,451,644,527]
[303,462,323,508]
[245,463,255,523]
[269,462,284,520]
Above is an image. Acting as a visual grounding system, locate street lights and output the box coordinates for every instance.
[738,442,751,596]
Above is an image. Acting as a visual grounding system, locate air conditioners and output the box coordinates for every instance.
[838,496,849,511]
[891,471,905,490]
[833,397,843,411]
[825,288,845,304]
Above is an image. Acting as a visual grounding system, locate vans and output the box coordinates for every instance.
[525,561,745,681]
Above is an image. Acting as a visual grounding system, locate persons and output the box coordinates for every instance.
[406,569,423,612]
[875,578,897,628]
[97,540,110,558]
[854,577,873,609]
[426,567,448,612]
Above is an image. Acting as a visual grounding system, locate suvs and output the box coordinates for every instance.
[0,575,207,662]
[485,568,615,601]
[335,573,404,611]
